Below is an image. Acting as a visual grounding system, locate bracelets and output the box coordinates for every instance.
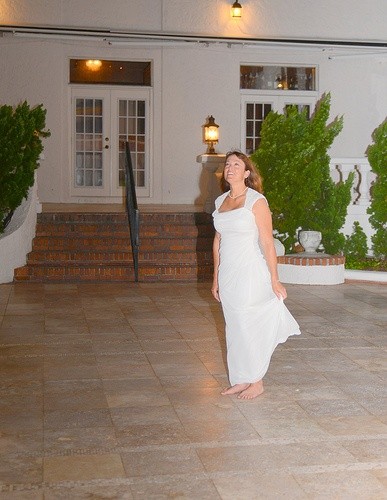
[272,280,280,286]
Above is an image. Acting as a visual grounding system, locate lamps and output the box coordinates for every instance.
[231,0,242,17]
[204,115,219,155]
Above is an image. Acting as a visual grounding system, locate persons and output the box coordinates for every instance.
[212,152,301,400]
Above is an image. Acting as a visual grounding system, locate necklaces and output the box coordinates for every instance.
[228,187,249,198]
